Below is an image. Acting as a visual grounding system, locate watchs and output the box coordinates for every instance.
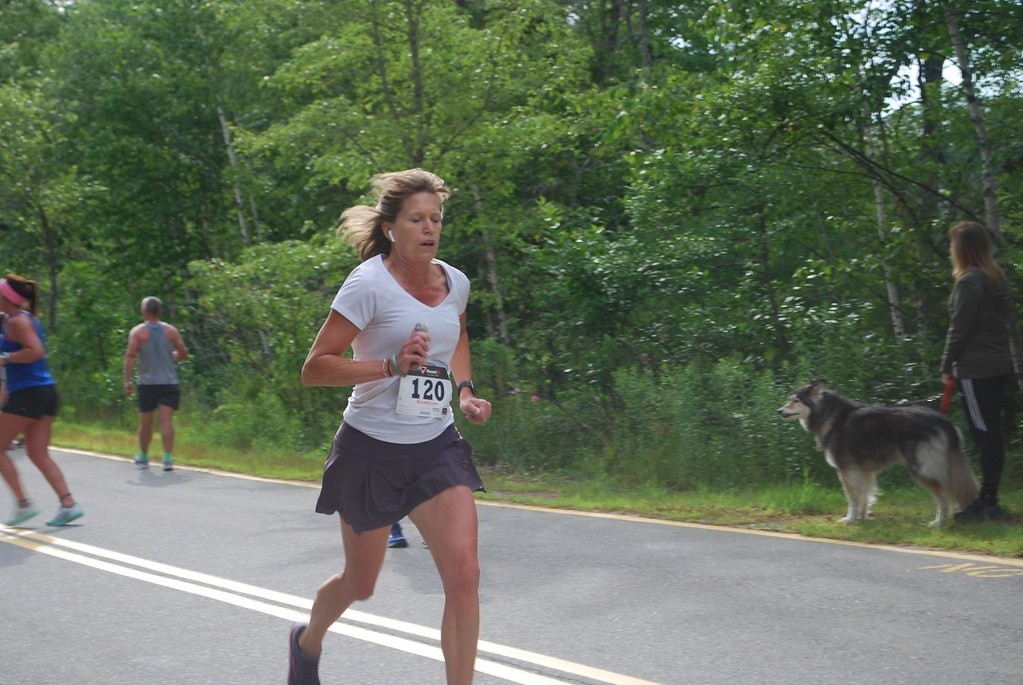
[457,380,476,396]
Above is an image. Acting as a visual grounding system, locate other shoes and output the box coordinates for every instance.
[288,625,321,685]
[954,497,999,523]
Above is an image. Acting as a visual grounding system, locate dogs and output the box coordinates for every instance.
[775,378,980,528]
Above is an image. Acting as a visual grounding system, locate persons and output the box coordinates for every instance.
[387,521,429,550]
[287,168,492,685]
[0,273,84,526]
[125,296,188,471]
[940,221,1013,524]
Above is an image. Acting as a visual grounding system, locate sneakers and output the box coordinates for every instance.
[386,524,406,549]
[135,455,148,468]
[7,504,36,526]
[46,504,84,526]
[163,460,172,471]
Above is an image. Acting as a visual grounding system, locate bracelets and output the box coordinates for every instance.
[383,354,406,378]
[1,352,10,365]
